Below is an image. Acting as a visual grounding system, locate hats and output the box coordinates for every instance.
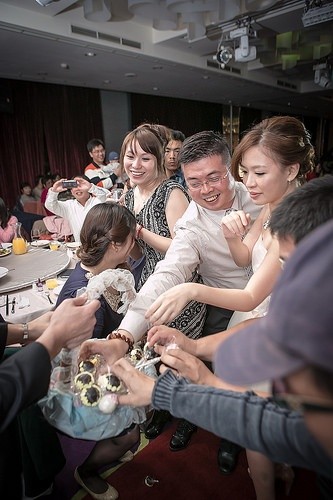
[213,220,333,386]
[108,151,119,160]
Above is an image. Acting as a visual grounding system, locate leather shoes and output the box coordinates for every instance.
[168,419,197,451]
[145,409,172,439]
[217,444,238,475]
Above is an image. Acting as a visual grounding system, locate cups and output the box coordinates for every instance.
[49,241,57,250]
[45,274,59,290]
[112,187,125,206]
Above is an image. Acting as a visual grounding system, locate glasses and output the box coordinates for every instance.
[91,148,105,153]
[272,378,333,413]
[185,166,232,191]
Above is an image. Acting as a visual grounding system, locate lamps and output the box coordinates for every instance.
[313,62,333,88]
[215,27,257,66]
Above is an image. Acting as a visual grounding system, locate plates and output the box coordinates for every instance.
[0,267,9,278]
[0,249,11,256]
[31,240,50,246]
[53,287,63,295]
[1,243,12,248]
[65,241,82,247]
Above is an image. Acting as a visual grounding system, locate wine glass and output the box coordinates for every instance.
[31,229,40,248]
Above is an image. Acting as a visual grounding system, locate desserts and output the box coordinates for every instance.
[75,357,122,406]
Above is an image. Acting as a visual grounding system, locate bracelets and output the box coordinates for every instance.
[107,333,133,356]
[20,323,29,346]
[136,226,143,240]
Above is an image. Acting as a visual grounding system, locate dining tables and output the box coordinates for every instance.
[0,241,81,319]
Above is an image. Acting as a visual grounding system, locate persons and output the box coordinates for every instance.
[0,117,333,500]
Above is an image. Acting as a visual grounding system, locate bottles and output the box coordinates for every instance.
[38,278,42,291]
[9,221,26,254]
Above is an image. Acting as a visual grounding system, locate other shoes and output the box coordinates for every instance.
[119,449,134,462]
[74,465,119,500]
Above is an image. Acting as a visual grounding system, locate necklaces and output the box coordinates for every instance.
[262,216,272,230]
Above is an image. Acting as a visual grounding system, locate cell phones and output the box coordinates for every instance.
[62,180,77,188]
[57,269,75,278]
